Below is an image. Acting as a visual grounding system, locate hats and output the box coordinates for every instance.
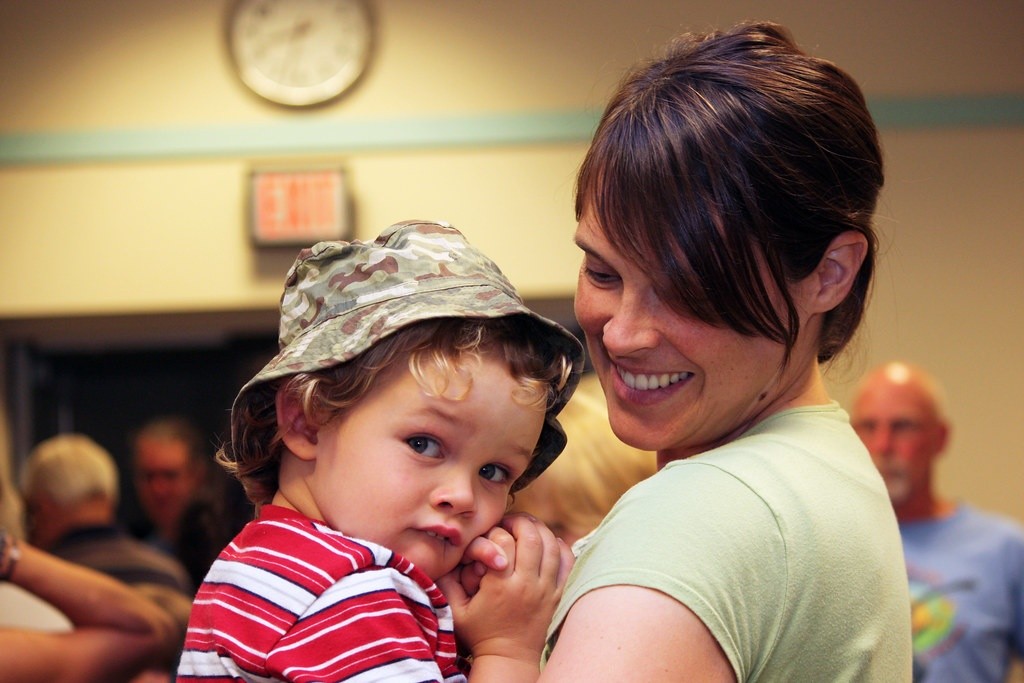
[28,434,117,512]
[230,219,586,495]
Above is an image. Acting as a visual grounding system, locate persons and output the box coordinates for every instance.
[849,361,1024,683]
[19,433,187,593]
[134,419,254,579]
[534,24,913,683]
[0,412,182,683]
[175,220,586,683]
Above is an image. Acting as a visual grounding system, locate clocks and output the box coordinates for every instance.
[220,0,379,111]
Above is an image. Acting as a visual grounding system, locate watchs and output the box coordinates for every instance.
[3,542,21,581]
